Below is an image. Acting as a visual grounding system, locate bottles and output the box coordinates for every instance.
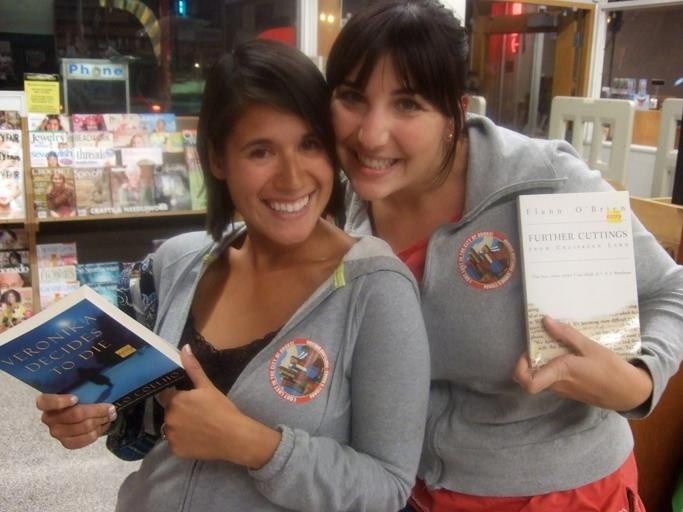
[601,78,664,110]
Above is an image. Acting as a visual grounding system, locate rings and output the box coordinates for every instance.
[159,423,167,441]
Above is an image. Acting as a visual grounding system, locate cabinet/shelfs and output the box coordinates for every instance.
[0,107,242,334]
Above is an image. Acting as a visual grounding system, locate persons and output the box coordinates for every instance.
[36,41,432,512]
[0,284,187,413]
[325,1,683,512]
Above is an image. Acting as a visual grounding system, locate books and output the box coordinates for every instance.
[516,191,641,378]
